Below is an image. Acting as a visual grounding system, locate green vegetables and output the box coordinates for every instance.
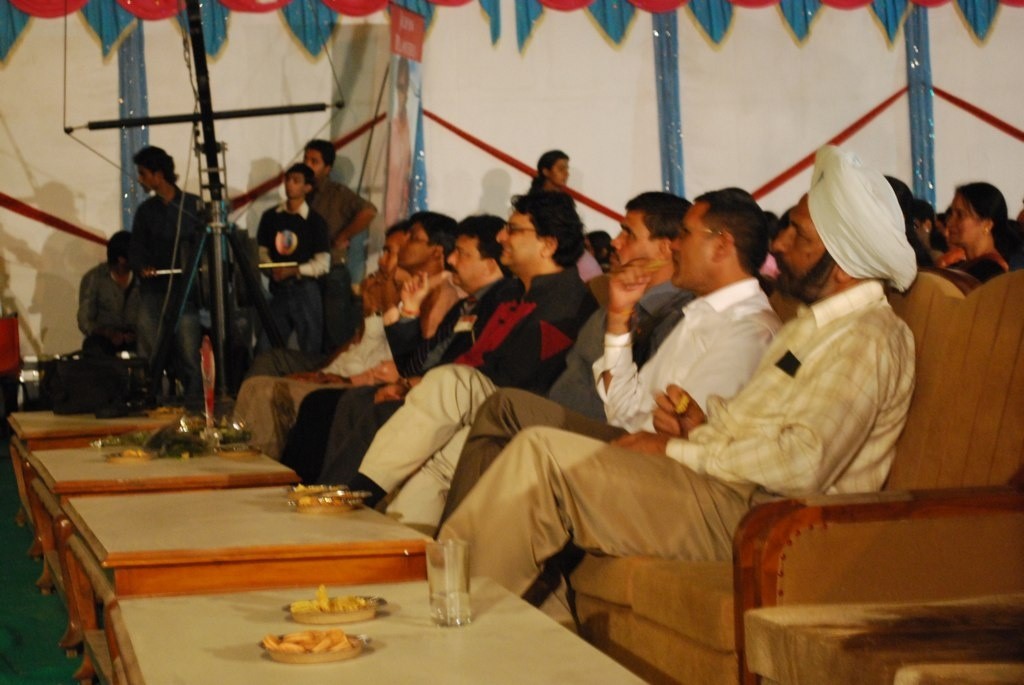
[94,419,249,457]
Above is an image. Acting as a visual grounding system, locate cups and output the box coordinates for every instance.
[426,540,474,627]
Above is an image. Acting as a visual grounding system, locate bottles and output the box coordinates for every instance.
[18,356,40,406]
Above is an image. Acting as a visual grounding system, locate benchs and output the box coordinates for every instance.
[555,269,1024,685]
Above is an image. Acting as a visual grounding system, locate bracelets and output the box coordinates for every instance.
[401,308,420,316]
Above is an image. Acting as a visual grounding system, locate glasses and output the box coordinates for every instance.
[504,220,540,235]
[676,222,725,237]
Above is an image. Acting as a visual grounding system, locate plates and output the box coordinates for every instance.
[283,483,371,512]
[258,632,374,664]
[282,595,388,625]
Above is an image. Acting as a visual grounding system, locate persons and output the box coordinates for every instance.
[303,139,377,354]
[233,181,1024,538]
[131,146,213,410]
[434,143,914,641]
[531,149,570,193]
[77,231,144,354]
[254,162,332,358]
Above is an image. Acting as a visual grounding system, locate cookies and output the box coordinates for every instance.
[261,628,352,653]
[675,394,689,413]
[288,584,369,613]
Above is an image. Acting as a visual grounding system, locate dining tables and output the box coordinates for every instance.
[7,411,652,685]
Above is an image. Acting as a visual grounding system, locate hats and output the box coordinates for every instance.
[810,144,918,291]
